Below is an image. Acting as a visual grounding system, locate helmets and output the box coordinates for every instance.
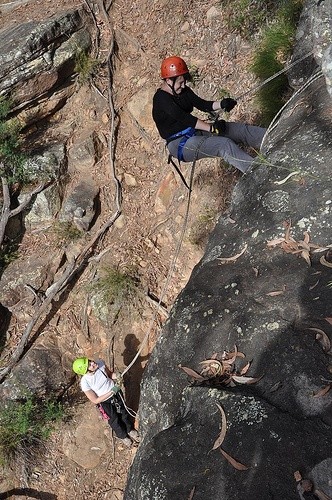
[160,55,194,83]
[72,357,88,375]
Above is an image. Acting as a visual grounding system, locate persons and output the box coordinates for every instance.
[152,56,268,174]
[72,357,140,447]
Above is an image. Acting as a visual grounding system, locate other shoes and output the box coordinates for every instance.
[121,430,141,447]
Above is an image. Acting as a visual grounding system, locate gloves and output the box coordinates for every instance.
[210,119,227,136]
[110,382,121,394]
[116,372,123,380]
[220,98,237,113]
